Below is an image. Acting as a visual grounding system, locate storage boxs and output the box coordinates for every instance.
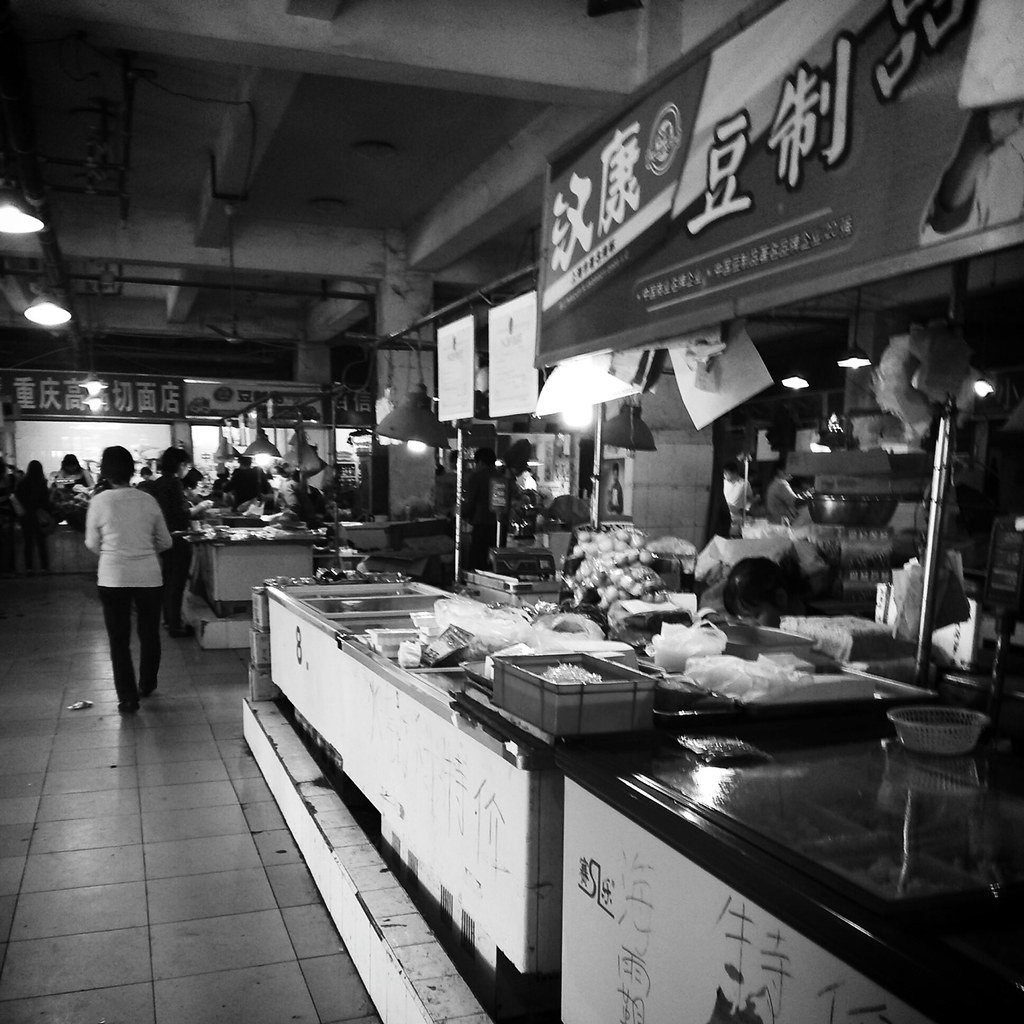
[784,450,930,493]
[490,653,660,738]
[249,627,271,671]
[248,663,273,701]
[221,516,265,528]
[251,593,269,634]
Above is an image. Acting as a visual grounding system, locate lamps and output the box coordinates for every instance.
[373,329,452,452]
[242,425,282,458]
[215,426,242,458]
[0,175,47,233]
[23,292,73,326]
[588,394,658,453]
[78,282,110,414]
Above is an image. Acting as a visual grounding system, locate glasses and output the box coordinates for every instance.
[186,465,192,470]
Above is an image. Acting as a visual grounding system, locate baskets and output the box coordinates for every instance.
[886,706,987,755]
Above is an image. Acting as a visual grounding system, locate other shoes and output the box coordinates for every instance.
[161,620,194,637]
[139,688,152,697]
[118,702,140,712]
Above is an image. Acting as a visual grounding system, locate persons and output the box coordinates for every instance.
[605,464,625,515]
[84,447,174,712]
[722,463,811,537]
[724,557,826,629]
[0,446,331,637]
[434,448,541,572]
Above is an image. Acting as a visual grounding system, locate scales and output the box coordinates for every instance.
[54,479,80,495]
[221,515,269,528]
[487,548,555,583]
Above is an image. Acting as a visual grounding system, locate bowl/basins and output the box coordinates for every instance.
[805,492,902,527]
[717,625,817,664]
